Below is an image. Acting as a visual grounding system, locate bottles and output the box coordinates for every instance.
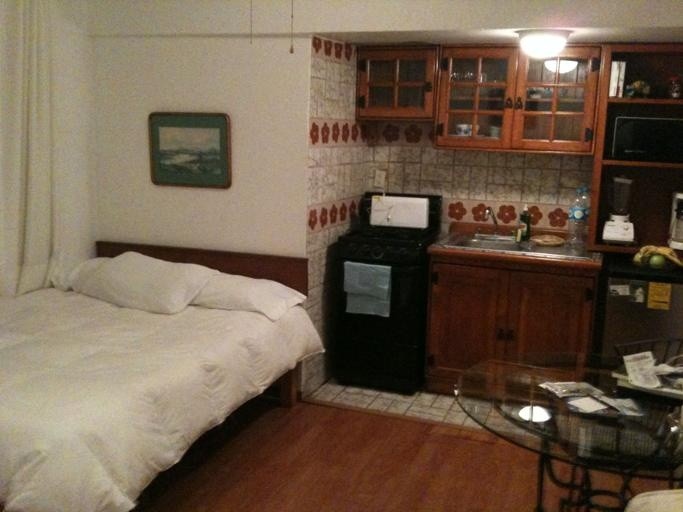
[667,76,681,99]
[567,189,586,256]
[520,204,530,241]
[581,188,590,240]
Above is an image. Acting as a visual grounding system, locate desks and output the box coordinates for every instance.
[453,346,683,512]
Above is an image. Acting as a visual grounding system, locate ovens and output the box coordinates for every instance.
[334,260,429,395]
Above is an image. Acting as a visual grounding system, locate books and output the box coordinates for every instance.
[610,363,683,399]
[608,60,627,98]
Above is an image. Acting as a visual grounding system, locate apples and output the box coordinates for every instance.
[649,254,666,270]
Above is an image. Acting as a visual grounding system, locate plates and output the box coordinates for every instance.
[531,235,566,247]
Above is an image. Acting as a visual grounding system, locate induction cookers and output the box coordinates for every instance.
[337,191,443,264]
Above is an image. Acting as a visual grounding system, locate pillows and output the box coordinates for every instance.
[81,250,219,314]
[189,272,308,322]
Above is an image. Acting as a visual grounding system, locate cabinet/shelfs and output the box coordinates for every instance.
[355,43,439,122]
[433,43,603,156]
[585,42,683,253]
[425,249,602,417]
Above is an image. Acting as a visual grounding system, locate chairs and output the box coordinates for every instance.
[613,337,683,379]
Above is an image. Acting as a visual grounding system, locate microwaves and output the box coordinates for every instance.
[606,113,683,164]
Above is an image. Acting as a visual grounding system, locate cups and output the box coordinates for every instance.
[490,126,500,137]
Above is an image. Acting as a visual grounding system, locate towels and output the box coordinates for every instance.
[343,261,392,318]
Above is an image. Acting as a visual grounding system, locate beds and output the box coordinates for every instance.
[1,241,327,511]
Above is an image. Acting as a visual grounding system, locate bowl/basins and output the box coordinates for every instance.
[456,125,480,136]
[527,87,546,99]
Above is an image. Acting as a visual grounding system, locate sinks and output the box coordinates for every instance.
[445,231,525,254]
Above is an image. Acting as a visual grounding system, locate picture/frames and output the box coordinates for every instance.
[148,112,233,189]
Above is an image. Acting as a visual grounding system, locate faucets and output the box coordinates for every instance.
[485,205,502,230]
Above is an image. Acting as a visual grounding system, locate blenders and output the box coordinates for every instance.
[667,192,683,251]
[602,177,634,246]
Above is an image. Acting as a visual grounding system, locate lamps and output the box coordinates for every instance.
[544,57,580,74]
[515,29,572,59]
